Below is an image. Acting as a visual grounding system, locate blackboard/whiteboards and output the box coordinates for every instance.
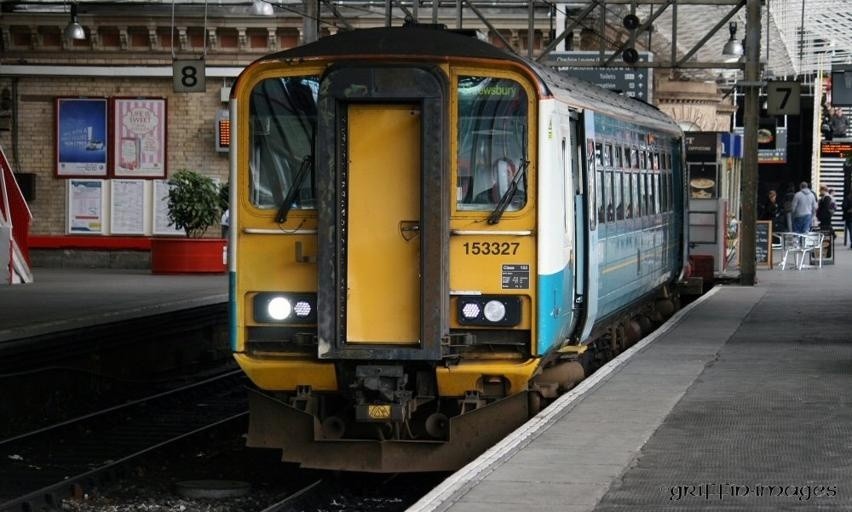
[754,220,772,266]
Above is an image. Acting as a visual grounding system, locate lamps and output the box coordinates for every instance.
[65,0,86,40]
[723,22,745,55]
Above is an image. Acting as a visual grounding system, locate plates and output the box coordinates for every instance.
[690,179,715,195]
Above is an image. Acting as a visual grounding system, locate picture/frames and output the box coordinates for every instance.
[109,95,168,179]
[67,179,102,236]
[152,179,192,236]
[55,98,108,178]
[110,179,145,235]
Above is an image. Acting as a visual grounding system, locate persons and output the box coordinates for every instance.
[829,108,848,138]
[842,190,852,250]
[821,107,836,141]
[472,156,527,211]
[763,178,839,258]
[819,85,835,117]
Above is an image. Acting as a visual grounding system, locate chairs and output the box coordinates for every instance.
[771,231,825,271]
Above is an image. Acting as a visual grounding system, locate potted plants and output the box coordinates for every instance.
[149,169,229,273]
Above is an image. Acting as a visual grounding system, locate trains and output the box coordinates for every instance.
[223,16,744,475]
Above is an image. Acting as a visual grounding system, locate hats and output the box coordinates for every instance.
[768,191,776,198]
